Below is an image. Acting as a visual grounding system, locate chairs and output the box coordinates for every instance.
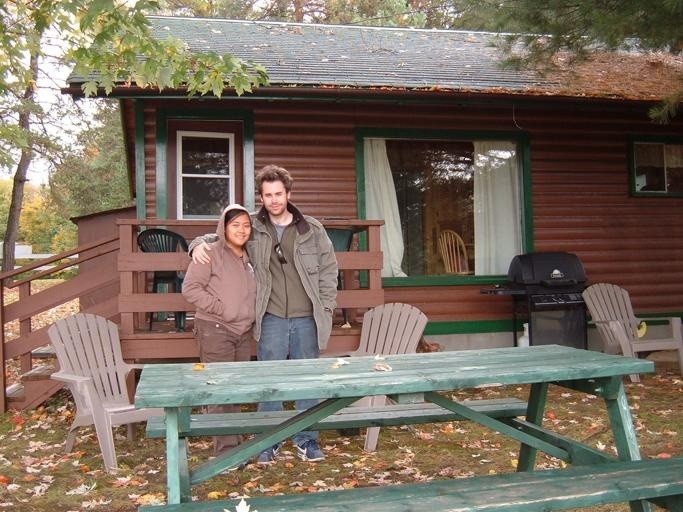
[45,311,163,473]
[582,282,682,384]
[338,300,432,451]
[323,218,357,330]
[136,230,193,333]
[435,229,473,275]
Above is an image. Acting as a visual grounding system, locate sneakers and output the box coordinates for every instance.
[225,445,279,471]
[296,440,325,462]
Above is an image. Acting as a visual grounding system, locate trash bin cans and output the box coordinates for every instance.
[481,251,594,349]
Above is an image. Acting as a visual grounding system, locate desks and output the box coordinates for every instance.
[133,344,654,512]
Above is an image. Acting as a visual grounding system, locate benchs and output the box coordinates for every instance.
[146,399,528,438]
[137,456,682,511]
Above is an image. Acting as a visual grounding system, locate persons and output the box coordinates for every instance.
[187,163,339,464]
[181,204,258,468]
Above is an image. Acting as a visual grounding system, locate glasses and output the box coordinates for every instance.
[273,243,287,265]
